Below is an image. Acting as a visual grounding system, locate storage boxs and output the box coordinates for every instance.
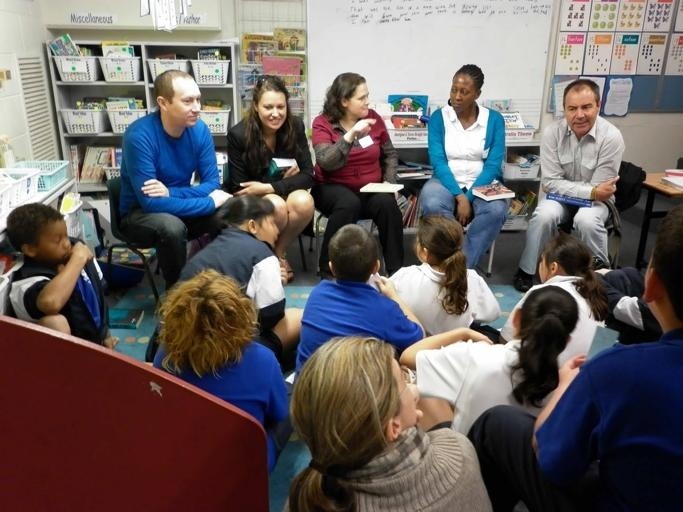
[97,57,141,83]
[264,55,302,84]
[107,109,148,135]
[59,105,107,135]
[102,163,122,183]
[52,56,98,82]
[59,200,84,243]
[192,105,232,136]
[0,168,41,219]
[0,253,31,314]
[147,56,190,84]
[189,58,230,84]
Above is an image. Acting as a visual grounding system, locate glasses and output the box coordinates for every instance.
[398,365,415,399]
[256,74,280,88]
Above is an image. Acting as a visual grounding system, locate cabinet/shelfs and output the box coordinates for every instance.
[239,44,308,124]
[391,137,539,234]
[45,37,239,193]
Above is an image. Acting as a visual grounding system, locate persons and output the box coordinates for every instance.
[295,223,427,380]
[513,80,626,292]
[153,267,295,480]
[311,72,405,284]
[591,266,662,345]
[281,336,494,512]
[117,69,233,291]
[224,75,314,282]
[145,195,304,365]
[6,202,120,349]
[419,64,509,270]
[465,204,683,512]
[479,235,612,369]
[387,214,502,335]
[396,285,579,440]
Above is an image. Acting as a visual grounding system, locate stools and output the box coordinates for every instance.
[104,242,160,305]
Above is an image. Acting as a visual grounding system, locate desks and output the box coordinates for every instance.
[634,173,683,272]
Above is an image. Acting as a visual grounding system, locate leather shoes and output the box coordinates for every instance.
[514,267,532,292]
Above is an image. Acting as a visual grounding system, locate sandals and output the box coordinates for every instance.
[279,254,295,281]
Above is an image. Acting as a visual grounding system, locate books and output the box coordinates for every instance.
[76,96,107,110]
[70,143,112,184]
[395,194,419,227]
[49,33,92,57]
[472,180,515,202]
[106,97,143,110]
[359,182,404,194]
[101,40,134,57]
[396,159,433,181]
[508,189,537,215]
[197,48,227,60]
[267,158,297,176]
[546,193,592,209]
[112,147,122,168]
